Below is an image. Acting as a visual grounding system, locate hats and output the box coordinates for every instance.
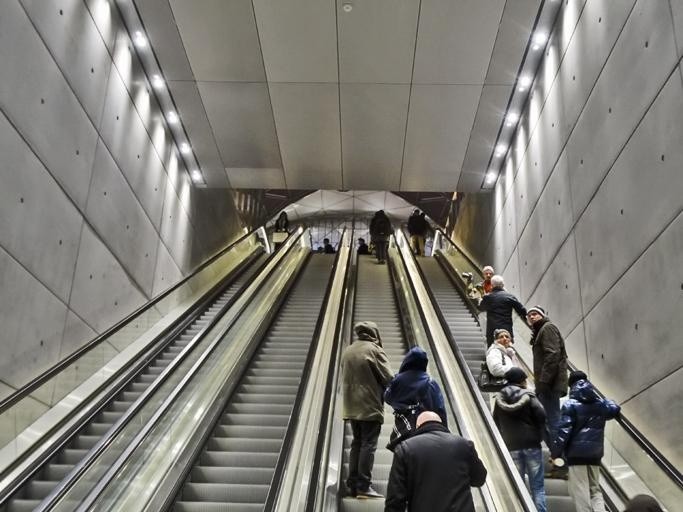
[528,305,546,319]
[567,371,587,386]
[494,328,512,340]
[505,369,527,384]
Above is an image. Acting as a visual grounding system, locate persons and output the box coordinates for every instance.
[341,320,395,498]
[317,210,434,265]
[472,275,529,344]
[525,305,567,476]
[382,346,445,436]
[465,267,496,336]
[625,493,662,511]
[275,209,288,247]
[382,409,488,511]
[491,366,549,512]
[484,328,528,387]
[550,370,620,511]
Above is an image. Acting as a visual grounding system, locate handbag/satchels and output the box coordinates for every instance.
[477,364,508,390]
[272,232,288,242]
[385,406,415,451]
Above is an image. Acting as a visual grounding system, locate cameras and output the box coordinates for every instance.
[462,272,472,283]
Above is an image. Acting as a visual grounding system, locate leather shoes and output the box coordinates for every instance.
[345,486,384,499]
